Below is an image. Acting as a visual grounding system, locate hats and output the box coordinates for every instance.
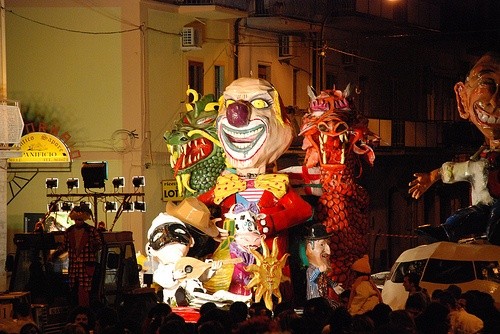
[71,205,96,222]
[351,255,371,274]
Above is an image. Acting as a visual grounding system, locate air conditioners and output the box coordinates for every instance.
[183,27,202,48]
[282,36,302,57]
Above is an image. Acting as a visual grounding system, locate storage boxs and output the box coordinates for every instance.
[0,291,32,321]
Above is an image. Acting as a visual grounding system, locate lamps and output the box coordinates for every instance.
[316,44,372,61]
[46,160,146,212]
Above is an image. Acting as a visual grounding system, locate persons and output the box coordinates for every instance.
[145,212,252,305]
[48,204,102,309]
[0,272,500,334]
[304,223,345,301]
[197,77,312,304]
[407,53,500,246]
